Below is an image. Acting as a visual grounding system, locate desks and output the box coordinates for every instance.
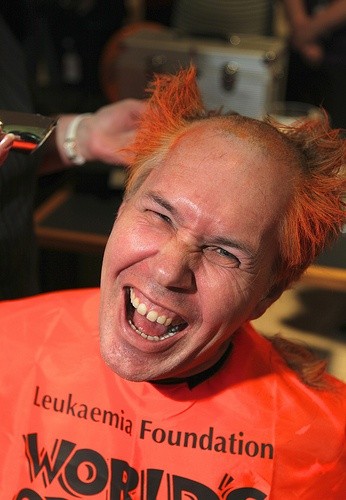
[32,161,346,294]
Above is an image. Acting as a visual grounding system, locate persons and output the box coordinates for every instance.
[281,0,346,142]
[0,94,151,303]
[1,60,346,500]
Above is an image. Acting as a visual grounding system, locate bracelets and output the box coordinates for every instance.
[61,111,89,168]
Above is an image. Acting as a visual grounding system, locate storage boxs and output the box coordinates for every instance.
[116,29,288,121]
